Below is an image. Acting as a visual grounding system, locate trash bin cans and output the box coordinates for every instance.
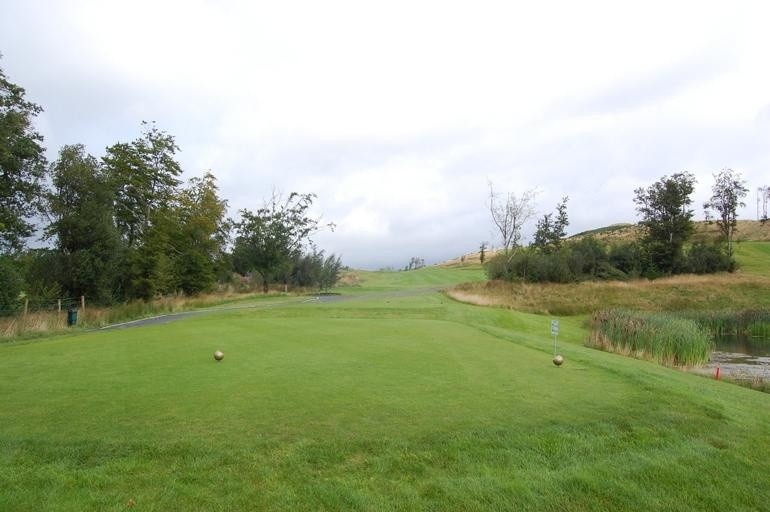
[68,310,77,325]
